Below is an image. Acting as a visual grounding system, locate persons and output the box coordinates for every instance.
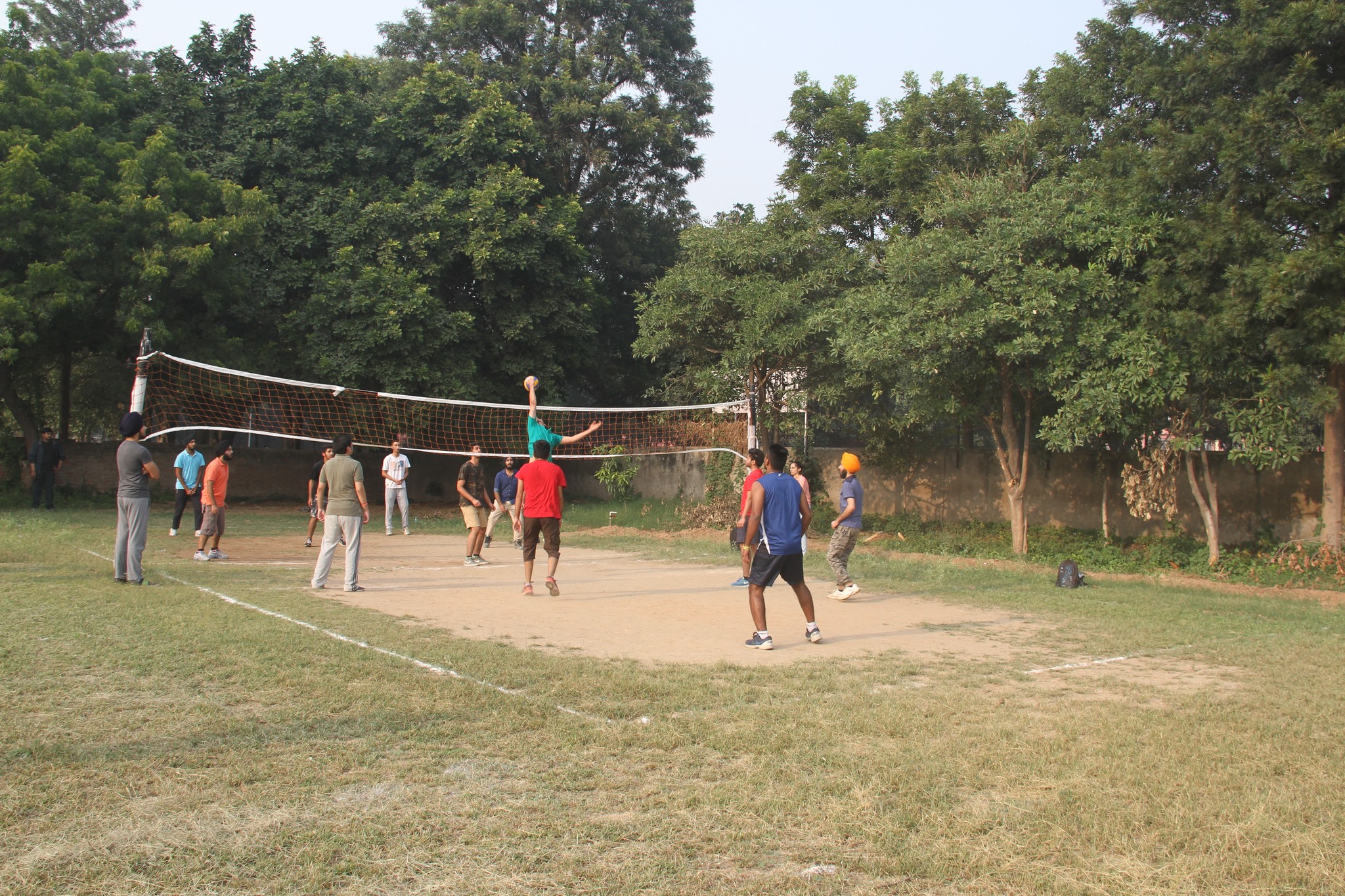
[169,434,205,537]
[483,457,526,549]
[826,453,863,600]
[382,439,410,535]
[29,426,65,510]
[741,444,822,650]
[194,440,234,561]
[790,461,811,562]
[115,411,161,587]
[311,434,370,592]
[305,444,347,547]
[512,440,567,596]
[730,448,765,586]
[456,442,490,566]
[525,377,602,464]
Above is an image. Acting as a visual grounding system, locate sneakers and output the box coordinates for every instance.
[401,526,410,535]
[521,581,535,595]
[463,557,479,566]
[194,551,210,561]
[208,550,229,559]
[838,584,860,600]
[304,537,312,547]
[514,539,524,550]
[744,632,774,650]
[827,586,844,599]
[730,576,749,587]
[805,626,822,642]
[339,536,346,546]
[385,529,393,535]
[472,555,489,564]
[483,535,492,547]
[545,576,560,596]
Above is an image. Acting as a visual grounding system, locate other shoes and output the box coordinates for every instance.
[195,529,200,537]
[344,586,364,592]
[46,509,54,512]
[130,578,151,586]
[169,528,177,536]
[113,578,130,585]
[317,585,325,589]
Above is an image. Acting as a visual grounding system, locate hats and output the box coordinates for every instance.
[119,411,143,437]
[214,440,230,456]
[841,452,861,473]
[184,434,196,446]
[503,457,515,465]
[42,427,52,434]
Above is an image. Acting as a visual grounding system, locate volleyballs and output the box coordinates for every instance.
[524,376,539,392]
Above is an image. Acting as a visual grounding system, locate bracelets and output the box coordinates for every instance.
[361,502,368,506]
[743,545,751,550]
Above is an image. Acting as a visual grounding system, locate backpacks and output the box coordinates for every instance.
[1055,560,1085,589]
[729,527,741,551]
